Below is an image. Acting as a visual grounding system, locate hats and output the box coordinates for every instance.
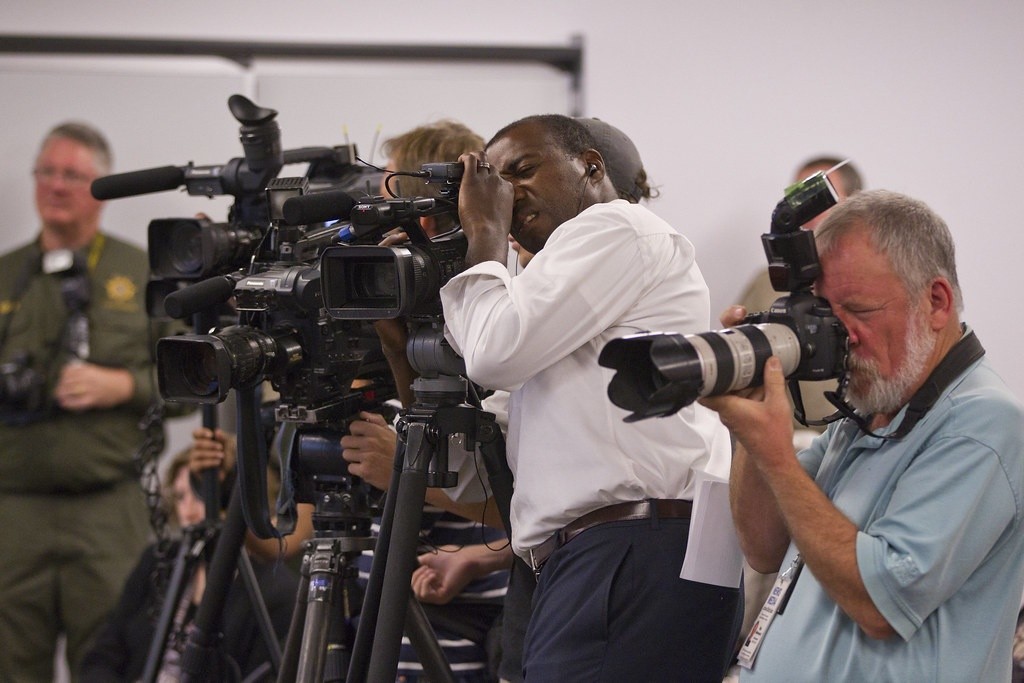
[573,117,642,202]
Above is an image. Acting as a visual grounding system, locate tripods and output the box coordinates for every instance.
[139,330,516,683]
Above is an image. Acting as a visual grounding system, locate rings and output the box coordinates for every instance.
[74,384,83,398]
[477,162,490,168]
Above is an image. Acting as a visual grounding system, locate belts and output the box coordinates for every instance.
[529,499,693,576]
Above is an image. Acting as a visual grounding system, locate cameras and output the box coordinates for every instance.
[599,171,854,424]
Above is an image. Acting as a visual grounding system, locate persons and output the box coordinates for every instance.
[697,188,1024,683]
[76,443,291,683]
[0,123,198,683]
[189,115,746,683]
[734,157,865,434]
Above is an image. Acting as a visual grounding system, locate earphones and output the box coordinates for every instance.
[587,163,600,177]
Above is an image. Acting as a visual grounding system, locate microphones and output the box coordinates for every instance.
[284,191,358,226]
[90,164,195,201]
[163,273,245,320]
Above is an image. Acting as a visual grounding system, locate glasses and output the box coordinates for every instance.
[32,164,90,188]
